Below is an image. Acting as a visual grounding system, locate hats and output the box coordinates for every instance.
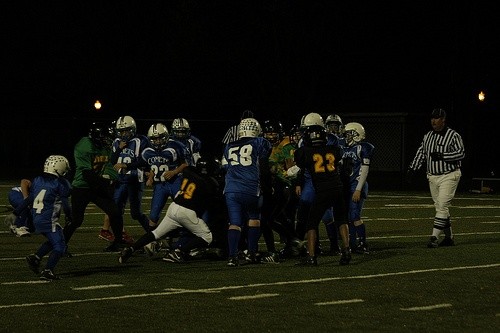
[431,108,446,120]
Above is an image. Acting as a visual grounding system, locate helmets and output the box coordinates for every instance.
[44,155,69,177]
[196,158,217,177]
[238,113,365,142]
[147,118,189,137]
[89,116,136,139]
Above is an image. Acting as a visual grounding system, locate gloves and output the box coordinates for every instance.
[431,152,444,161]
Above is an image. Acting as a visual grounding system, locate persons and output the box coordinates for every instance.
[7,113,375,279]
[405,108,466,248]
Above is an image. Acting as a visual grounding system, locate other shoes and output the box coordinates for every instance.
[26,253,41,273]
[39,267,61,281]
[9,225,31,237]
[427,236,438,248]
[97,228,370,267]
[439,235,456,246]
